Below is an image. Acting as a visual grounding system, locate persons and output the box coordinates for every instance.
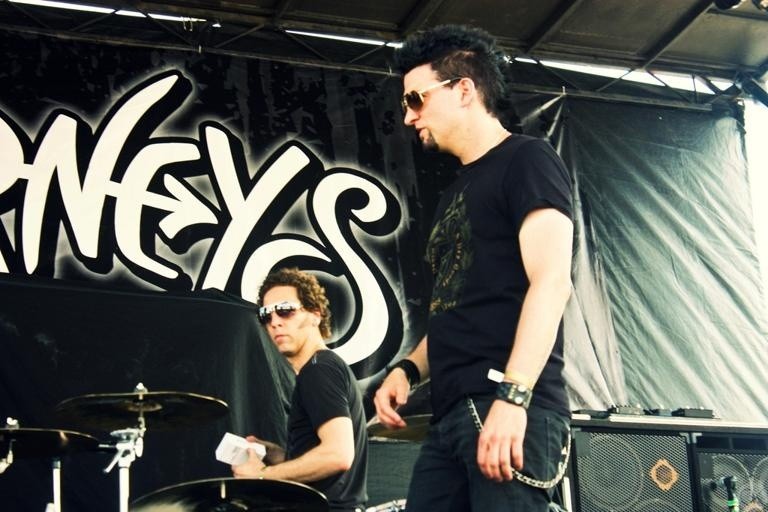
[227,266,370,512]
[371,21,581,510]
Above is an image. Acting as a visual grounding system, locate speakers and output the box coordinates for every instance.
[569,427,698,512]
[691,431,767,512]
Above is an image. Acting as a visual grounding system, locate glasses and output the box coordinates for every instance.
[399,78,461,110]
[257,301,302,325]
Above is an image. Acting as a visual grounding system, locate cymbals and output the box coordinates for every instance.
[366,377,432,431]
[0,427,97,464]
[53,389,230,446]
[367,414,433,443]
[128,476,331,512]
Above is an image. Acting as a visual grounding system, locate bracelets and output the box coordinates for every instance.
[258,465,270,480]
[385,358,421,391]
[494,380,533,410]
